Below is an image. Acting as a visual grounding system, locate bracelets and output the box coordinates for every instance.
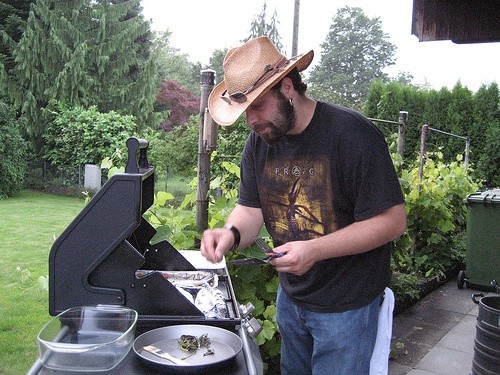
[225,225,241,253]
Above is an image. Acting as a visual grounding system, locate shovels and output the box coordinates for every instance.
[227,255,276,266]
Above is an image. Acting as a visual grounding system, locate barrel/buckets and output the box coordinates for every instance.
[471,292,500,375]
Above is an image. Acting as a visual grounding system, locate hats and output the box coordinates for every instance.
[208,36,314,126]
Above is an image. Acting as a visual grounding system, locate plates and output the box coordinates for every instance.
[132,324,243,373]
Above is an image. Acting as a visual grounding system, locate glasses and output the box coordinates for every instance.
[220,68,273,104]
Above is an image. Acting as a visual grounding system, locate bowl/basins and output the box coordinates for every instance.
[36,304,139,372]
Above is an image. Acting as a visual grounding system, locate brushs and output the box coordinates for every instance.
[143,345,189,365]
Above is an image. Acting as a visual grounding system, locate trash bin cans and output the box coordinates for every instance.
[458,191,500,290]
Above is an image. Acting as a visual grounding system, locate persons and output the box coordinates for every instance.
[199,35,406,375]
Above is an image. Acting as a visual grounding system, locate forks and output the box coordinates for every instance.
[253,237,283,259]
